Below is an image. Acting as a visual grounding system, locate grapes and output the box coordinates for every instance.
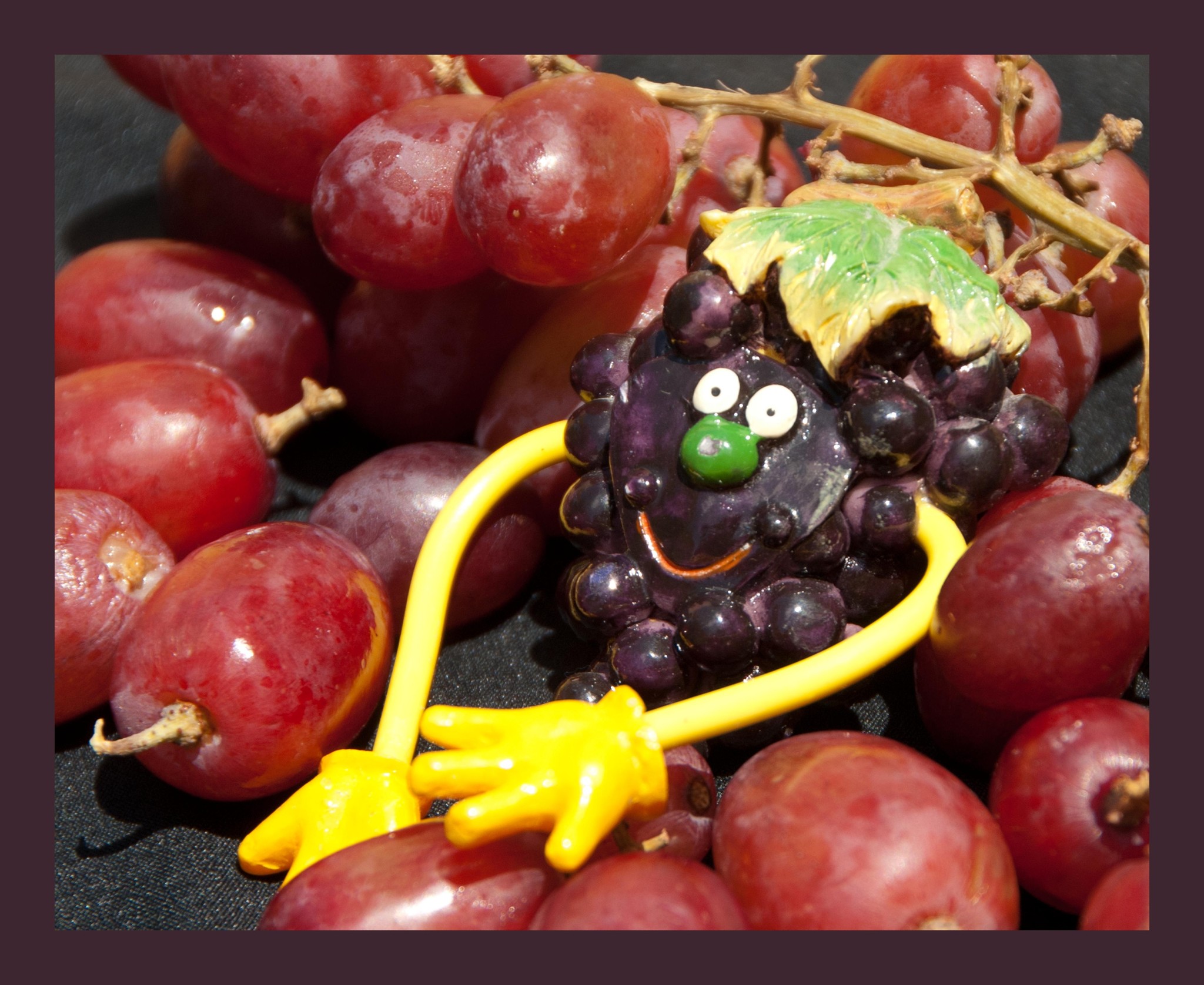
[55,55,1151,932]
